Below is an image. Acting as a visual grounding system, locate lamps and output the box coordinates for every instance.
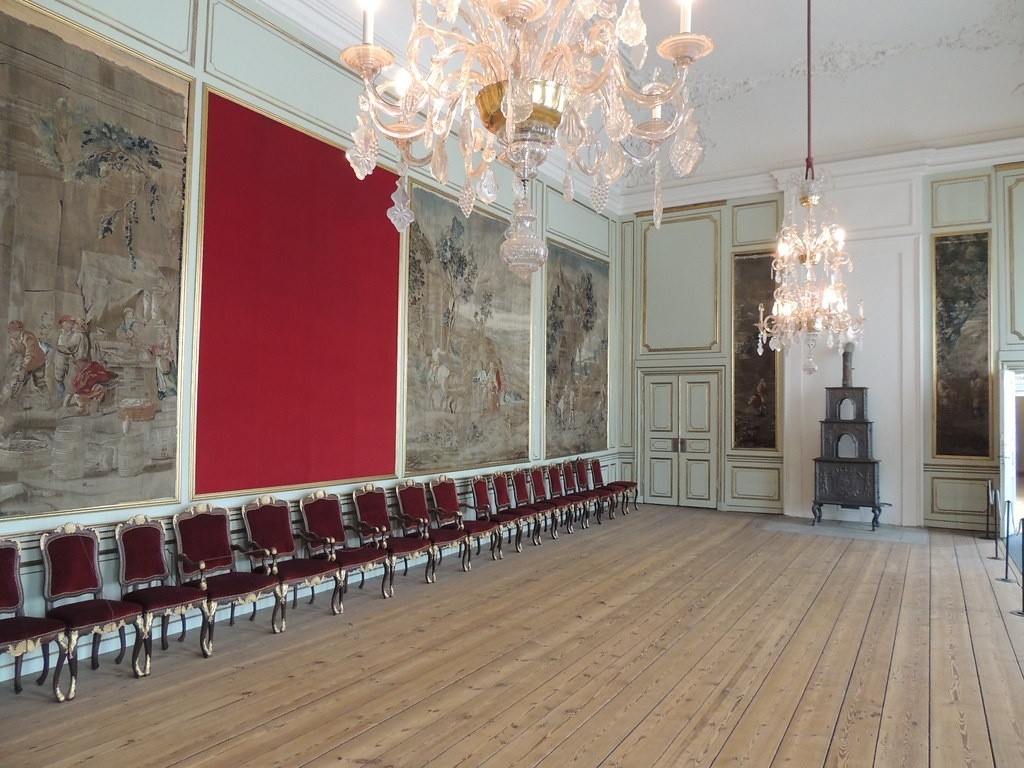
[338,0,715,276]
[755,1,865,356]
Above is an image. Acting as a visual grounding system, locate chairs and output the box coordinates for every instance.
[0,456,639,704]
